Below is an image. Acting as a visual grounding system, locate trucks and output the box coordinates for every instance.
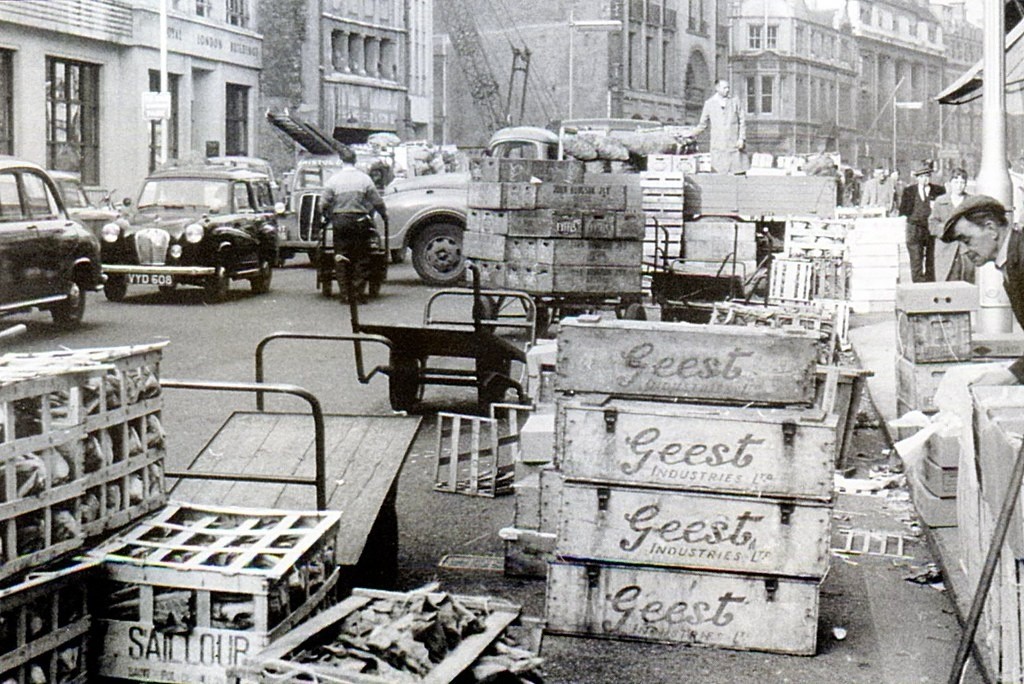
[386,122,838,288]
[269,142,396,266]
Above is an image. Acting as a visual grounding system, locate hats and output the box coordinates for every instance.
[940,195,1006,244]
[915,166,934,176]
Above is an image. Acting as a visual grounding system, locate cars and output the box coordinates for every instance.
[36,171,128,267]
[0,158,109,332]
[103,161,287,306]
[196,157,282,215]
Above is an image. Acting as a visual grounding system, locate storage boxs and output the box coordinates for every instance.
[686,217,758,261]
[464,160,645,297]
[518,315,836,656]
[892,282,1024,684]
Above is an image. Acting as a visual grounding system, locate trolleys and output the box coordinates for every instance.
[349,279,539,417]
[153,331,426,587]
[315,210,389,300]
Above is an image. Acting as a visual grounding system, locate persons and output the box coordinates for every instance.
[368,143,459,193]
[684,79,750,176]
[938,194,1024,386]
[798,145,904,218]
[898,166,946,283]
[319,150,390,305]
[929,168,976,286]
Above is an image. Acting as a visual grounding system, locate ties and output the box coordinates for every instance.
[922,185,927,200]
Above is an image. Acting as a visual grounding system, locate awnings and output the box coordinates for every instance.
[935,18,1024,115]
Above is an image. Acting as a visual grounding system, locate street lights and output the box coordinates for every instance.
[568,11,624,124]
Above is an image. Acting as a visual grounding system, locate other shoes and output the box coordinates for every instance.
[340,297,349,303]
[354,291,368,305]
